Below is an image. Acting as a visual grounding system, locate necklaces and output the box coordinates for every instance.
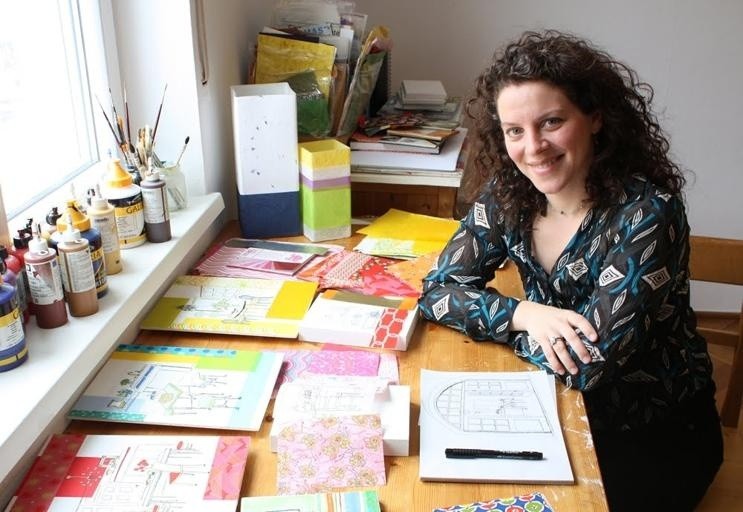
[552,205,583,215]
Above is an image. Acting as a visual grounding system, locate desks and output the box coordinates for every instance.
[353,182,457,219]
[11,219,609,510]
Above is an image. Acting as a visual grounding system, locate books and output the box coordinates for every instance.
[227,248,314,276]
[419,368,575,485]
[369,49,472,178]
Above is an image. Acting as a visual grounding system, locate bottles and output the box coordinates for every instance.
[0,159,188,373]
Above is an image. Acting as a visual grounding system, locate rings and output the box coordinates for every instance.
[549,337,562,345]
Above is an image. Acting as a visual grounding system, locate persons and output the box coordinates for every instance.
[417,28,723,512]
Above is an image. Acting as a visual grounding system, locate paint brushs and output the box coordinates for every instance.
[94,80,191,209]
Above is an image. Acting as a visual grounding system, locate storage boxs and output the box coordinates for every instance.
[233,81,300,237]
[300,138,352,243]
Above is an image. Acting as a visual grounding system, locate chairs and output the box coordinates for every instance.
[686,233,743,512]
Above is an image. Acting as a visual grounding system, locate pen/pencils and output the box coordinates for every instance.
[445,449,541,460]
